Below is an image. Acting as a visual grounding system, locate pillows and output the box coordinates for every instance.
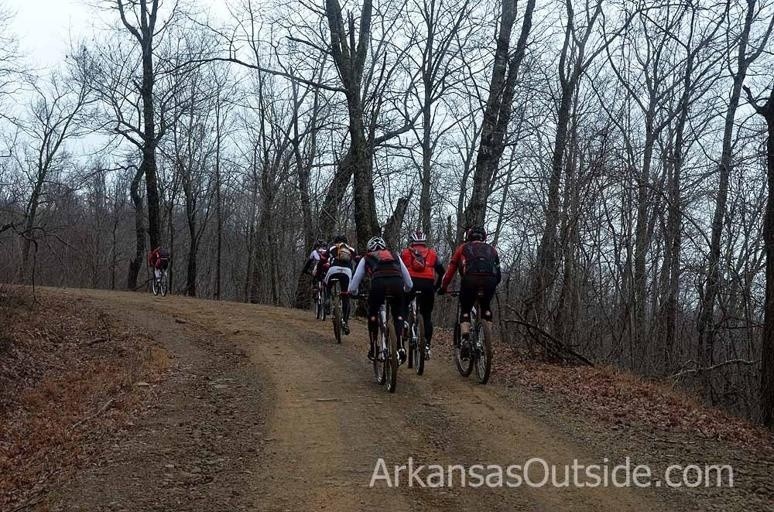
[315,239,327,247]
[408,231,427,246]
[463,225,487,242]
[335,234,347,243]
[367,236,387,252]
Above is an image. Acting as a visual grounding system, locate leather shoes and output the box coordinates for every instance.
[438,289,445,295]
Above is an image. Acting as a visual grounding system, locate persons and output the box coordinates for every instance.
[437,224,502,360]
[400,230,446,361]
[148,244,170,287]
[348,235,414,365]
[312,234,360,336]
[302,238,330,315]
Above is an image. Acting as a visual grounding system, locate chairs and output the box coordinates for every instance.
[340,319,350,335]
[368,352,377,361]
[460,338,473,361]
[396,348,407,366]
[425,345,431,360]
